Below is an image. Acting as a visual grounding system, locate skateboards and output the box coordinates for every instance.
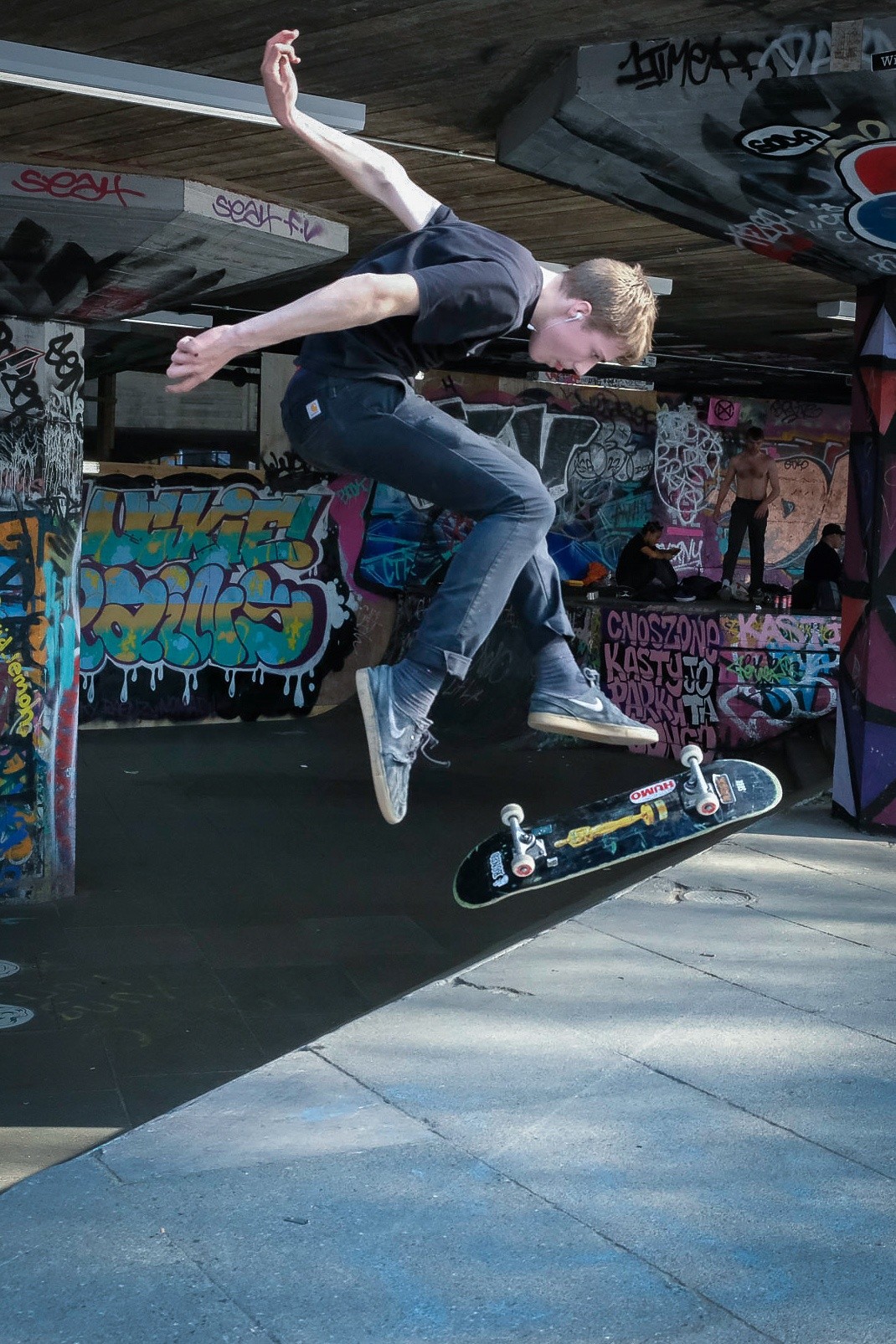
[453,744,783,907]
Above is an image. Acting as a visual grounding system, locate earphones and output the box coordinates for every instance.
[568,311,583,321]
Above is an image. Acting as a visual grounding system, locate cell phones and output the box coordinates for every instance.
[672,549,681,555]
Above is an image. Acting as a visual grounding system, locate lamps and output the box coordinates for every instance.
[817,299,856,322]
[121,311,213,330]
[538,262,673,295]
[0,39,366,134]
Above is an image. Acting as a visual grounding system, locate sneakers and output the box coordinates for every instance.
[528,668,659,745]
[355,664,450,825]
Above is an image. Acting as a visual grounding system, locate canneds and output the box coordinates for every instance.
[781,595,791,609]
[774,596,779,608]
[606,574,612,586]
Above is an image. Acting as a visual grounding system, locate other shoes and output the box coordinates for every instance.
[673,590,696,602]
[722,579,729,587]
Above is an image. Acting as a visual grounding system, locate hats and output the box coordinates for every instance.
[822,523,845,535]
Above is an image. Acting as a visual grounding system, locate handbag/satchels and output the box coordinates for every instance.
[719,584,750,602]
[792,579,826,610]
[679,576,722,600]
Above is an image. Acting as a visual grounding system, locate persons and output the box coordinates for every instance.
[801,523,847,585]
[711,426,780,611]
[165,27,659,826]
[616,521,697,602]
